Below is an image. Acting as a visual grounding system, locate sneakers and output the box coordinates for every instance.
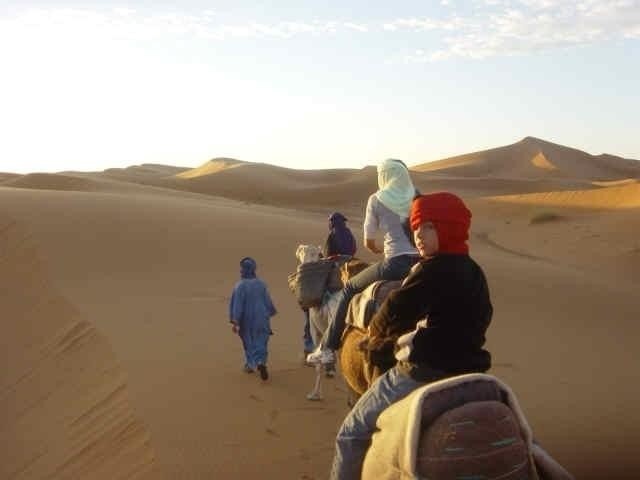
[306,345,334,363]
[245,363,268,381]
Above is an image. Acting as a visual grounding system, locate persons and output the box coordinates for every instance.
[230,255,278,382]
[321,211,358,258]
[301,309,338,378]
[304,158,422,365]
[332,190,494,479]
